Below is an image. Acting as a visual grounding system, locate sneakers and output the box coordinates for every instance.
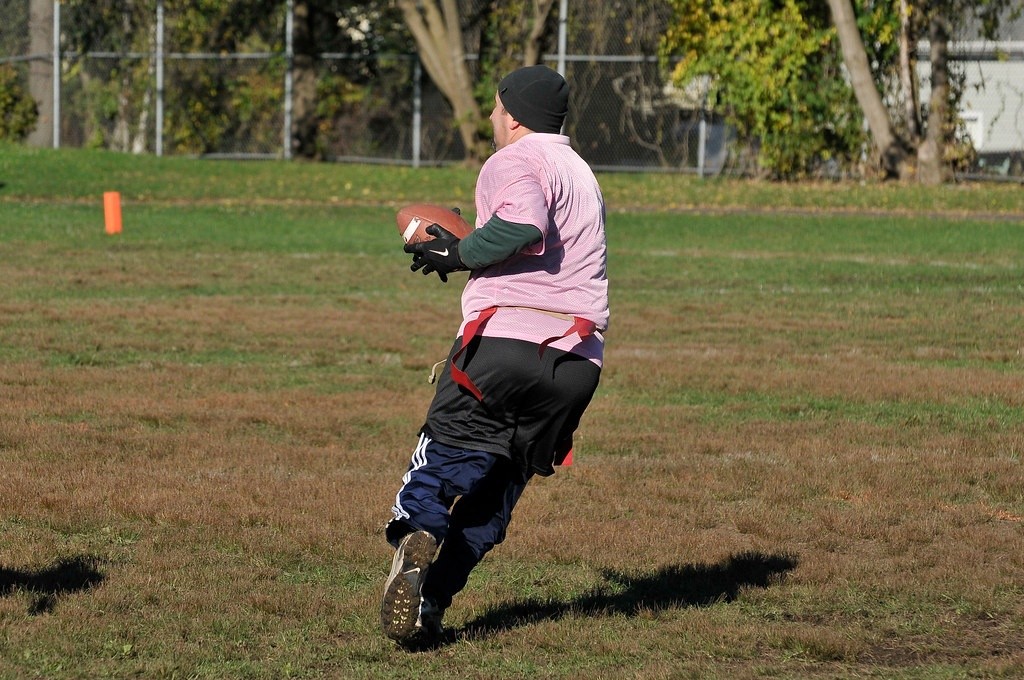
[380,530,437,641]
[415,593,450,628]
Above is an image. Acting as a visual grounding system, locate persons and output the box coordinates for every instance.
[380,63,608,649]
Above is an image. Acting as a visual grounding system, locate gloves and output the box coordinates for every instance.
[403,223,471,283]
[451,208,461,215]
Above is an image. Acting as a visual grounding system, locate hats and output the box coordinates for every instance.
[498,65,572,134]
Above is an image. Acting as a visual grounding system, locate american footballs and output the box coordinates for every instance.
[396,204,474,262]
